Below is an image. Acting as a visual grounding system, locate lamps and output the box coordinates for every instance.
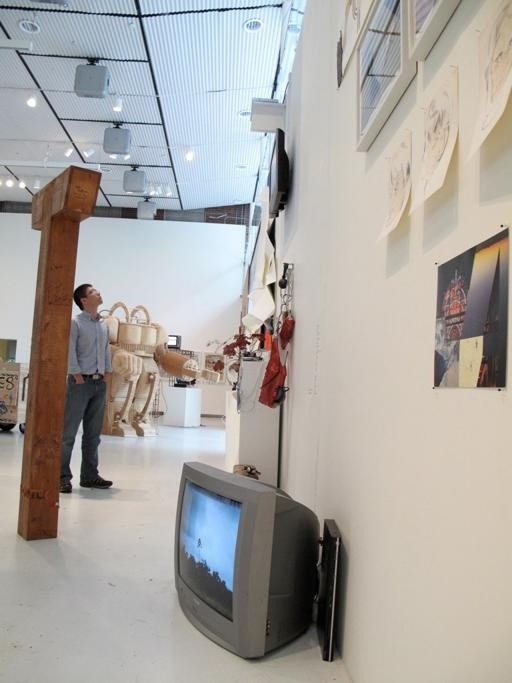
[74,58,157,220]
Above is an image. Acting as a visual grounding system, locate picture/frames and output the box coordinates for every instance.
[341,0,462,152]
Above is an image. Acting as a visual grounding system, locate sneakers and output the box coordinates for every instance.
[60,476,72,492]
[80,475,113,488]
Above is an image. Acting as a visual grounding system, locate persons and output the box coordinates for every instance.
[59,282,114,492]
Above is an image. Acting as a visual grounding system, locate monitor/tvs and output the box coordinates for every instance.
[173,460,321,660]
[267,127,290,219]
[167,334,182,349]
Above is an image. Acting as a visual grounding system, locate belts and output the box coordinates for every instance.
[83,374,102,380]
